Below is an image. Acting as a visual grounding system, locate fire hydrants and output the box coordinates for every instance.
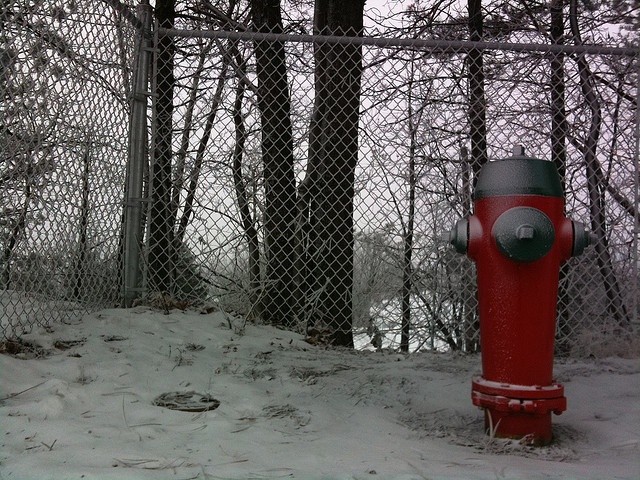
[441,146,598,446]
